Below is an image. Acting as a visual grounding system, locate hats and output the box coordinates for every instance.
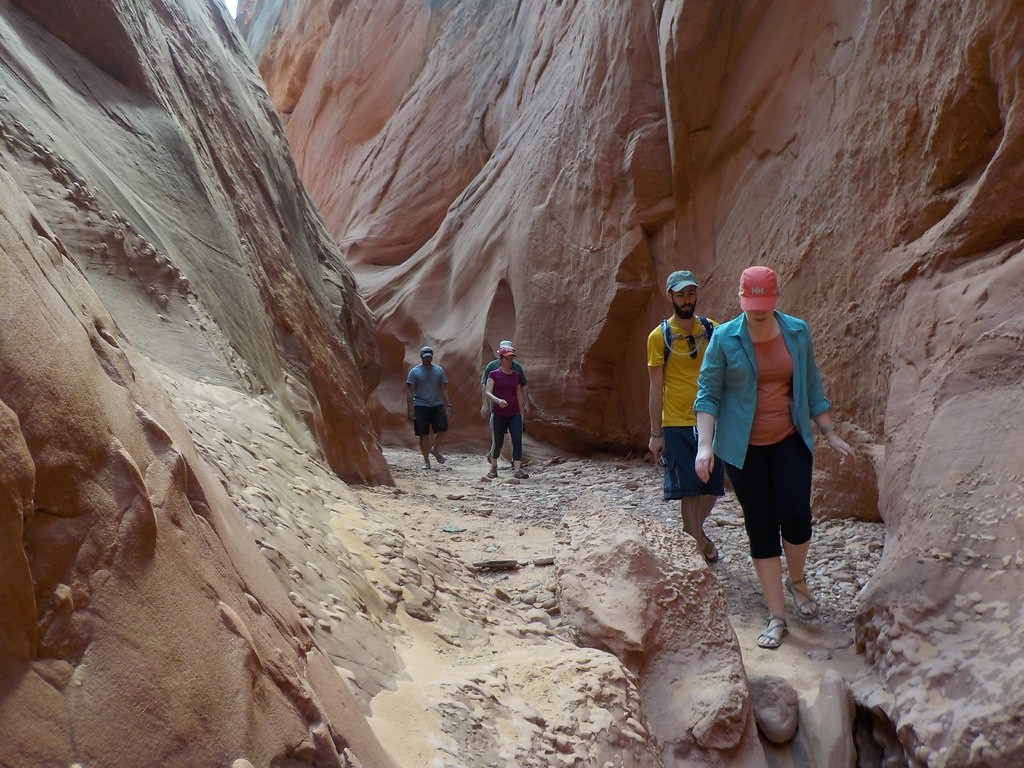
[420,346,432,358]
[497,348,516,358]
[739,266,778,310]
[499,341,514,349]
[666,270,698,292]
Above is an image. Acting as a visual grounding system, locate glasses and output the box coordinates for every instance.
[686,335,697,359]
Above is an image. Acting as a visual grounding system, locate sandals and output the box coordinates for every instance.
[700,531,719,564]
[757,613,789,649]
[785,575,818,619]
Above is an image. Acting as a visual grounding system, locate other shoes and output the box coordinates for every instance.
[431,447,446,463]
[514,470,529,478]
[487,467,498,478]
[421,463,429,469]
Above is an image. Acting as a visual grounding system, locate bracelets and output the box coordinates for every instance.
[651,433,663,438]
[821,425,835,435]
[446,404,452,408]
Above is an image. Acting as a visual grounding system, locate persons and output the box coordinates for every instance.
[481,341,528,480]
[694,266,857,649]
[406,346,453,469]
[647,270,726,560]
